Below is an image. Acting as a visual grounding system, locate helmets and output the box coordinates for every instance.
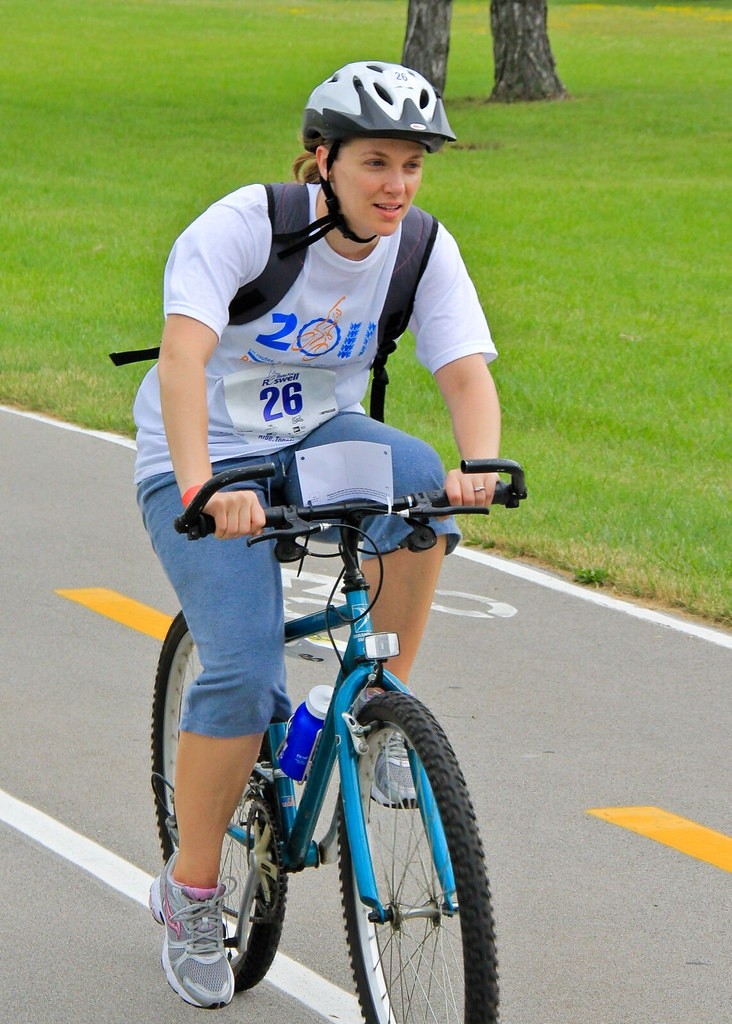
[302,61,457,155]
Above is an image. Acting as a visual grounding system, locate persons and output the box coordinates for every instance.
[130,56,509,1007]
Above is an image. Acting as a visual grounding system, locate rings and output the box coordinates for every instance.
[473,486,486,492]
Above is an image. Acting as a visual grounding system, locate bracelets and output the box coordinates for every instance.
[180,484,204,507]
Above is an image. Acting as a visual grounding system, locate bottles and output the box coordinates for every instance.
[278,686,330,783]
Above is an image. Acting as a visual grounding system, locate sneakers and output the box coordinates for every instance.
[150,850,237,1009]
[352,689,424,811]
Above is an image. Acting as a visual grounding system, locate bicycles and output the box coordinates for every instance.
[144,456,530,1024]
